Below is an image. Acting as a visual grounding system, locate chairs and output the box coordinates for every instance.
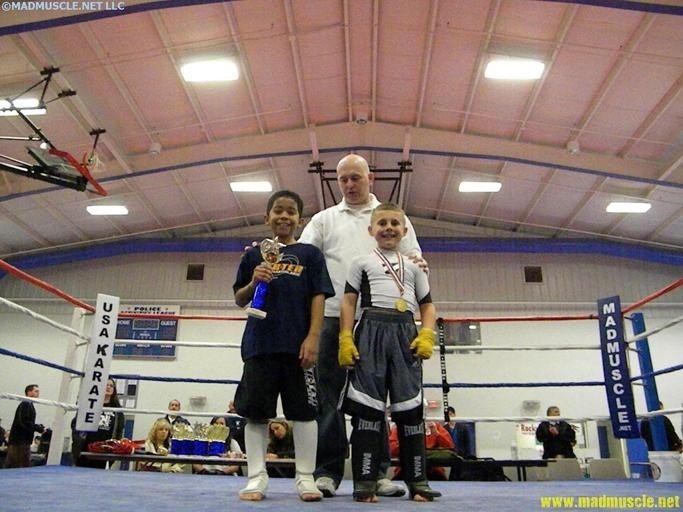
[547,457,582,480]
[587,457,628,481]
[502,460,537,480]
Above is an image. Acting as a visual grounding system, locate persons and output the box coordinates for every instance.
[230,189,335,501]
[266,417,294,479]
[387,398,454,480]
[337,201,435,504]
[163,398,189,451]
[192,415,243,476]
[224,398,246,476]
[639,401,681,451]
[70,375,124,469]
[535,406,576,459]
[240,154,429,497]
[2,383,44,468]
[136,418,187,473]
[442,407,475,481]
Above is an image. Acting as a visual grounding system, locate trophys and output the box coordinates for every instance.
[244,235,286,320]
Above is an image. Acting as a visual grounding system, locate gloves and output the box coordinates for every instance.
[409,327,438,361]
[337,329,361,369]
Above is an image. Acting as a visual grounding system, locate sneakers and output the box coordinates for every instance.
[374,477,406,499]
[315,476,337,499]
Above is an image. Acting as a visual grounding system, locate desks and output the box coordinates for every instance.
[77,445,296,477]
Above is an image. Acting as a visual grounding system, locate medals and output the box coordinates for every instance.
[395,298,406,313]
